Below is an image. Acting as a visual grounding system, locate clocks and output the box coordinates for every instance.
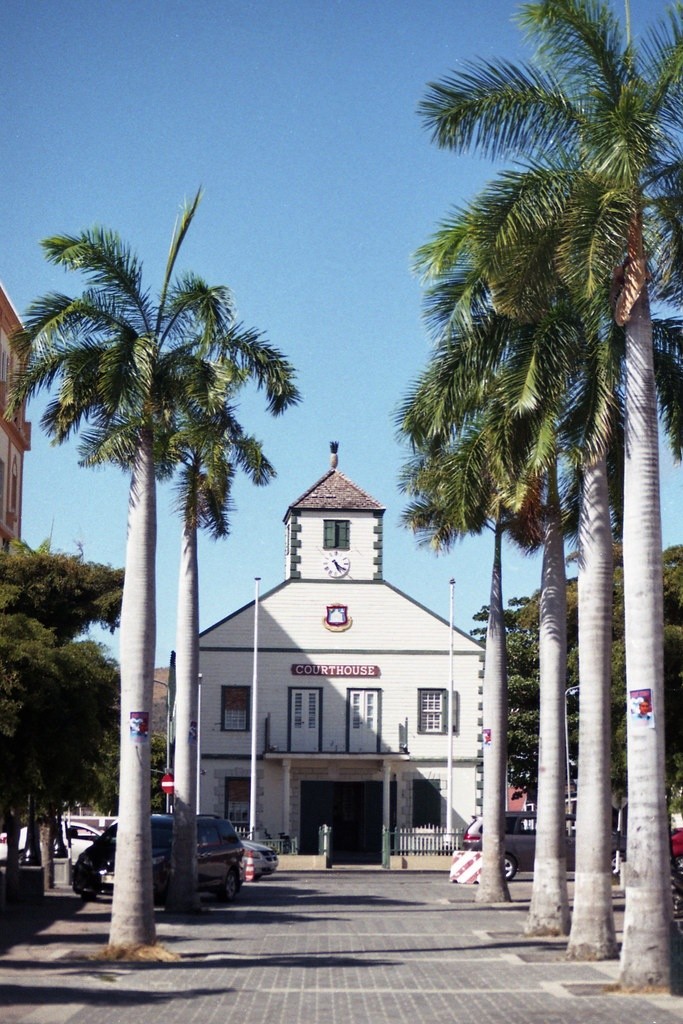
[323,550,350,578]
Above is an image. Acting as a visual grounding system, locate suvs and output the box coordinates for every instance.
[464,811,627,881]
[71,813,246,907]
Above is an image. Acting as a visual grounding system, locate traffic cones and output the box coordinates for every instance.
[246,850,255,880]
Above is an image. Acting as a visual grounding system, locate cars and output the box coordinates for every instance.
[0,820,103,868]
[241,841,279,881]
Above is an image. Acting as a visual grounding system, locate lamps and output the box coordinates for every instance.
[400,742,407,753]
[267,743,276,752]
